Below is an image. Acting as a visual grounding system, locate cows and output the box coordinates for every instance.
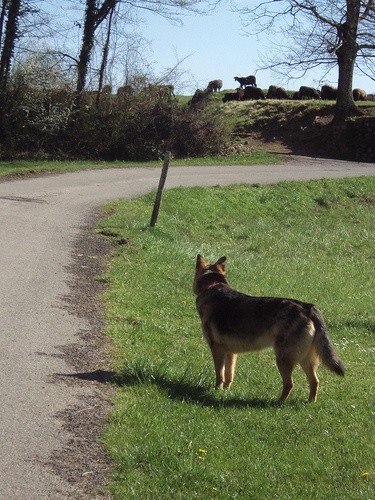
[208,80,222,92]
[233,75,257,89]
[159,84,176,98]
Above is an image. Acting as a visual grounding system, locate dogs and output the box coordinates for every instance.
[193,255,346,404]
